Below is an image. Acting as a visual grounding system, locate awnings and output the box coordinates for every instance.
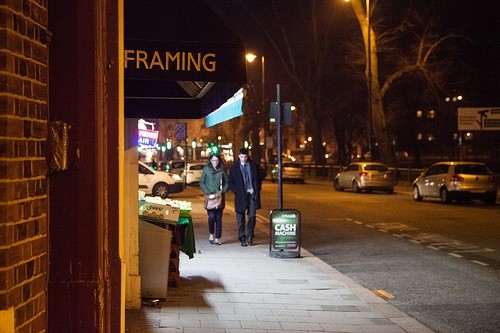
[124,0,247,128]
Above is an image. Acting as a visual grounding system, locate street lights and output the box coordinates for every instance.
[246,53,267,159]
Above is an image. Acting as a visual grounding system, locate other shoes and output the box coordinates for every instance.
[209,234,213,244]
[240,238,248,246]
[247,235,254,246]
[214,237,220,244]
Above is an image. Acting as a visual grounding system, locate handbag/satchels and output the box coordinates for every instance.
[204,191,225,210]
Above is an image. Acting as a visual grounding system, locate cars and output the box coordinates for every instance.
[170,161,185,173]
[271,161,305,184]
[411,161,497,206]
[333,162,395,194]
[160,162,167,170]
[138,161,184,199]
[181,163,208,186]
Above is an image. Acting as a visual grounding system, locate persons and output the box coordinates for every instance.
[199,153,229,245]
[229,148,267,247]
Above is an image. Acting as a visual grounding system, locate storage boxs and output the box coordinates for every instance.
[168,225,182,285]
[139,201,181,223]
